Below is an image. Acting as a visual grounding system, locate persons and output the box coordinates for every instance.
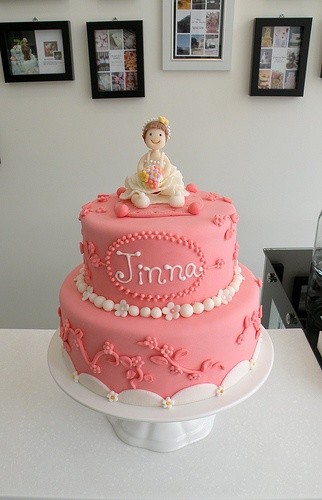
[118,116,190,208]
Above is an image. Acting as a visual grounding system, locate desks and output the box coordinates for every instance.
[0,328,322,500]
[259,248,322,369]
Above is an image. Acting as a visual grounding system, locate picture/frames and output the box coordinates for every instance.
[162,0,233,70]
[249,18,312,97]
[86,20,146,99]
[0,20,74,83]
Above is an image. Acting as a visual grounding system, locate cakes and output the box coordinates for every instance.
[57,116,263,408]
